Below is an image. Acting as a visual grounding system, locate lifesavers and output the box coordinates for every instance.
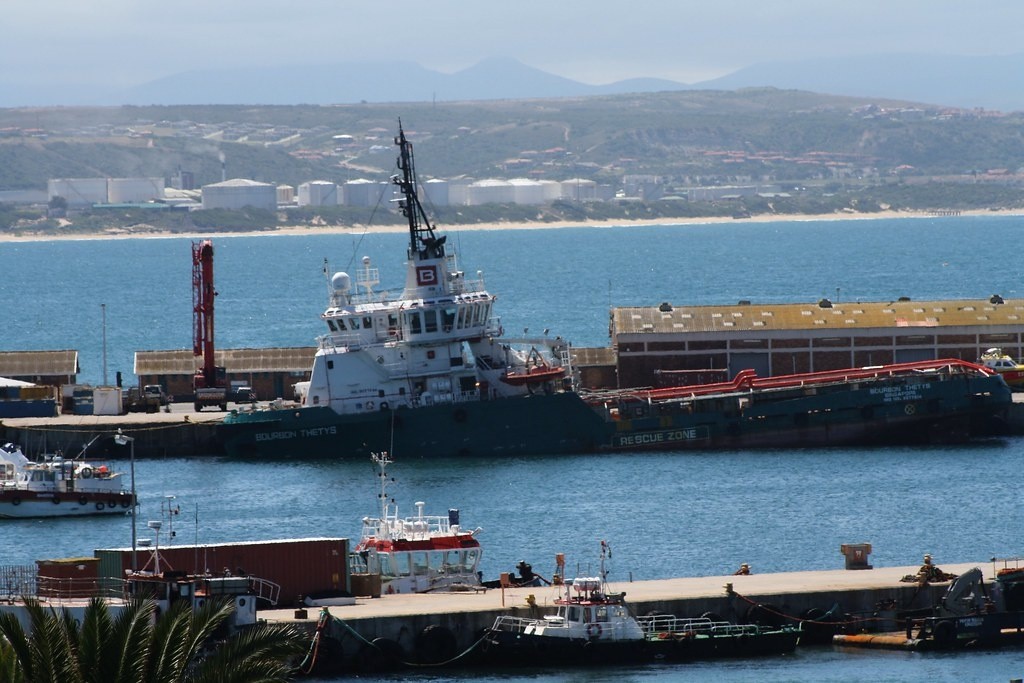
[12,498,22,505]
[96,501,105,510]
[108,498,117,508]
[121,500,130,507]
[587,622,602,640]
[387,414,403,429]
[79,496,88,505]
[452,406,469,423]
[81,468,92,479]
[53,495,61,505]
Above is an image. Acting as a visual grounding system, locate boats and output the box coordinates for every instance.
[480,561,804,667]
[977,346,1024,387]
[0,406,139,517]
[0,117,1012,464]
[348,451,540,593]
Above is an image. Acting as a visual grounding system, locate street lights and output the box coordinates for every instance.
[114,434,137,588]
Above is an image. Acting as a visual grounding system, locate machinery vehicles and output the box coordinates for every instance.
[191,240,226,411]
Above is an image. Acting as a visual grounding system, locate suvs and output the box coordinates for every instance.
[234,387,256,403]
[144,384,166,404]
